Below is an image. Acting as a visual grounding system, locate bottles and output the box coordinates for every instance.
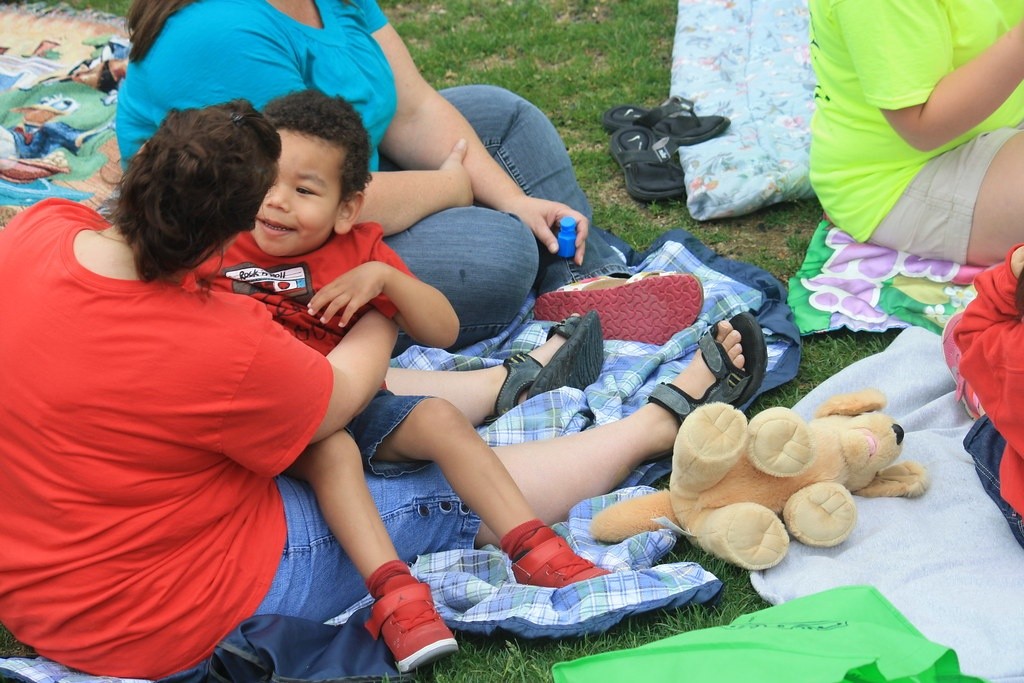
[558,217,577,257]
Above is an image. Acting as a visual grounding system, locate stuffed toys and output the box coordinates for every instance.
[591,387,930,571]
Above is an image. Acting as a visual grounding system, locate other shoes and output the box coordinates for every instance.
[942,310,983,421]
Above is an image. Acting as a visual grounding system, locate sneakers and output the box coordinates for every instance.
[512,533,612,589]
[365,574,458,674]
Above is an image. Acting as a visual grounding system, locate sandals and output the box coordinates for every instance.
[483,308,604,426]
[609,125,685,201]
[604,97,730,144]
[533,268,705,345]
[647,312,767,467]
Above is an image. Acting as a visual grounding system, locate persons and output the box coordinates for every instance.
[0,98,768,683]
[181,88,613,673]
[116,0,703,345]
[809,0,1024,267]
[941,244,1024,551]
